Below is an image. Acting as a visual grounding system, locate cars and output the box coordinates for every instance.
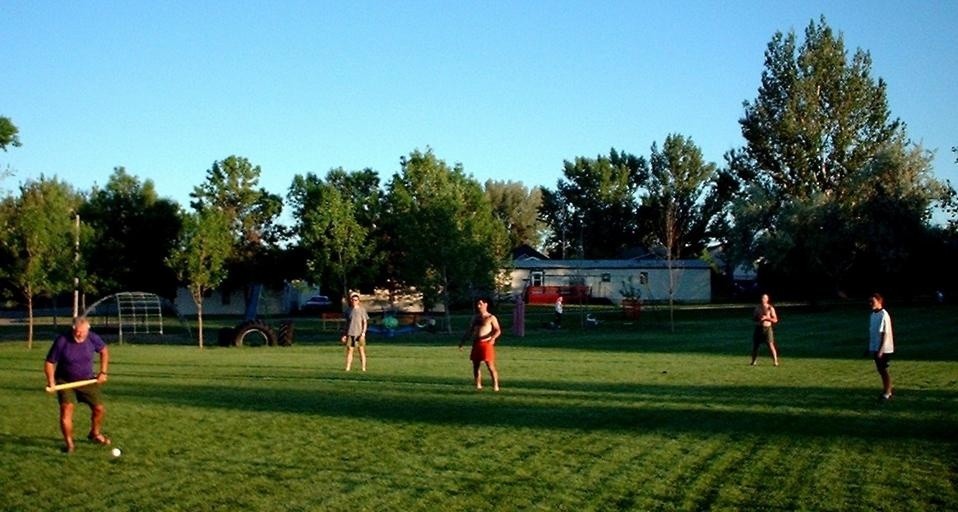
[305,296,333,308]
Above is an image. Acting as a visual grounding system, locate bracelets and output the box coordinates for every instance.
[361,333,366,336]
[101,371,107,375]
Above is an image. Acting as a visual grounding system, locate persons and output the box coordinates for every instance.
[44,317,111,454]
[550,297,563,328]
[340,295,370,371]
[459,297,501,391]
[750,293,778,366]
[868,293,895,400]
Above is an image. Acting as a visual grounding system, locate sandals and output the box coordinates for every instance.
[88,430,111,446]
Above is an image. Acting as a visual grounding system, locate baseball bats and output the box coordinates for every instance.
[45,377,106,394]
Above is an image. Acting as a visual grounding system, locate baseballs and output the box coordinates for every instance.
[111,448,120,457]
